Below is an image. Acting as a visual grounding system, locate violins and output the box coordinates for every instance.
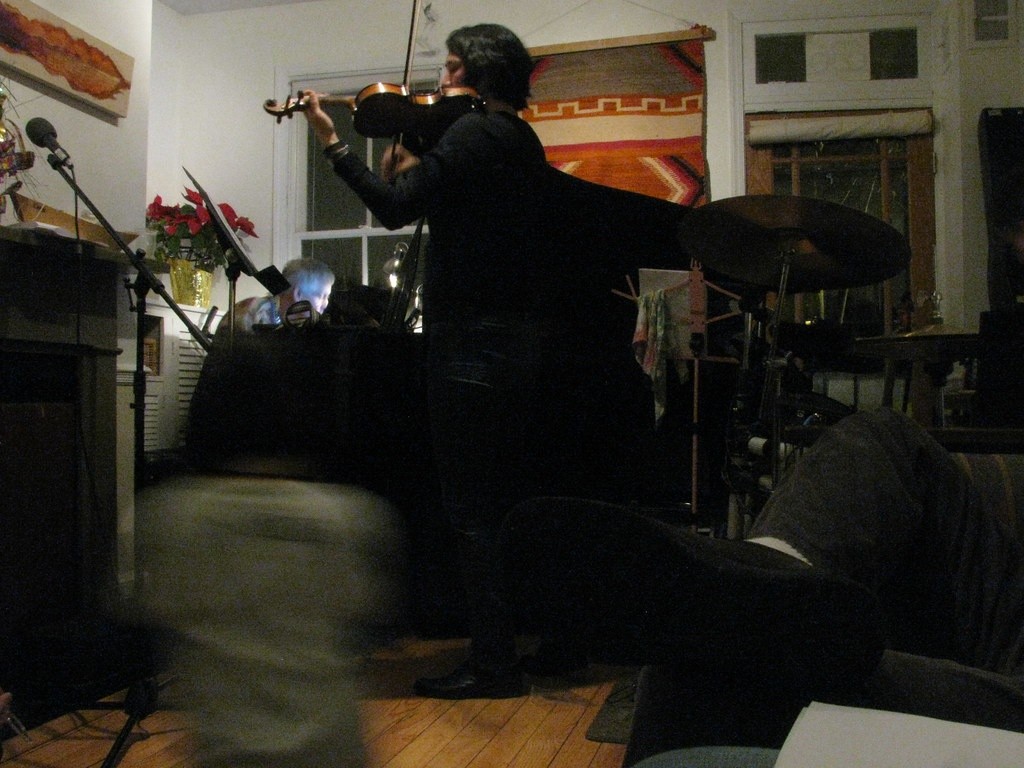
[261,84,480,137]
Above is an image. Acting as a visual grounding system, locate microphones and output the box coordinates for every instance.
[26,117,74,170]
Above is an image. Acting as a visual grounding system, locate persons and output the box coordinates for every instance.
[99,22,637,768]
[501,407,1024,756]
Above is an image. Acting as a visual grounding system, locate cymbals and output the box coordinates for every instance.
[676,193,913,293]
[854,320,981,362]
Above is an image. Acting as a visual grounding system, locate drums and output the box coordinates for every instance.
[759,318,855,447]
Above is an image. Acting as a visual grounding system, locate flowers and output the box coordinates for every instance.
[0,140,24,213]
[146,191,259,274]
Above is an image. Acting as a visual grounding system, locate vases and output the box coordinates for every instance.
[170,259,214,309]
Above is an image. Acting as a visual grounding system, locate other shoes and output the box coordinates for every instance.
[495,491,889,685]
[520,647,596,675]
[413,664,563,700]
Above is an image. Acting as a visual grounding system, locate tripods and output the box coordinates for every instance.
[45,153,217,768]
